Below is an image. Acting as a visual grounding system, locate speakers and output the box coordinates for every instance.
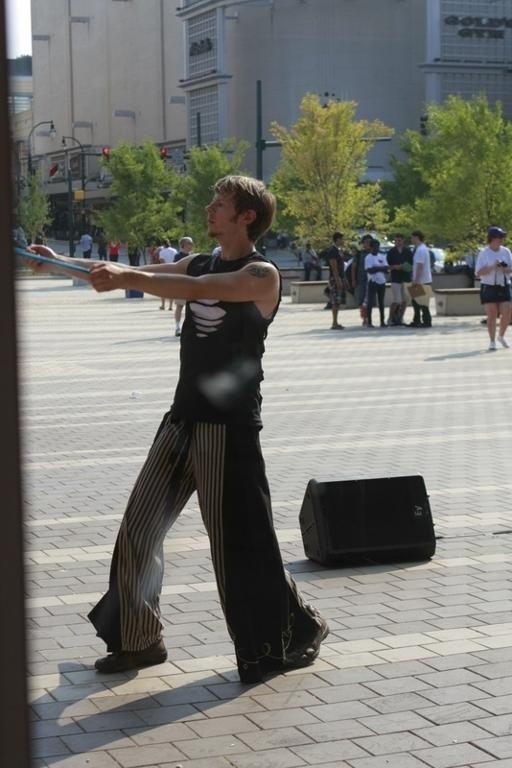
[296,472,437,568]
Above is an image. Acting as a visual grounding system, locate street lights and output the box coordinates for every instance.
[61,133,89,240]
[27,119,58,174]
[7,139,23,221]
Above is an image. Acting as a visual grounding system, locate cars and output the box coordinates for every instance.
[273,227,472,279]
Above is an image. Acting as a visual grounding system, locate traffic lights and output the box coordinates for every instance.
[160,147,168,163]
[418,113,432,136]
[101,146,111,165]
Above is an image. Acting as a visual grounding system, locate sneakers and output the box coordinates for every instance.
[293,615,329,668]
[489,336,510,350]
[176,328,182,336]
[95,636,167,672]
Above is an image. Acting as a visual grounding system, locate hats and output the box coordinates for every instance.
[487,226,506,237]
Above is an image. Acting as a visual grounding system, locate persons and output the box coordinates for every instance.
[172,235,195,338]
[24,174,331,684]
[296,229,511,331]
[146,229,222,330]
[106,235,121,261]
[96,233,109,260]
[79,231,94,258]
[474,225,512,351]
